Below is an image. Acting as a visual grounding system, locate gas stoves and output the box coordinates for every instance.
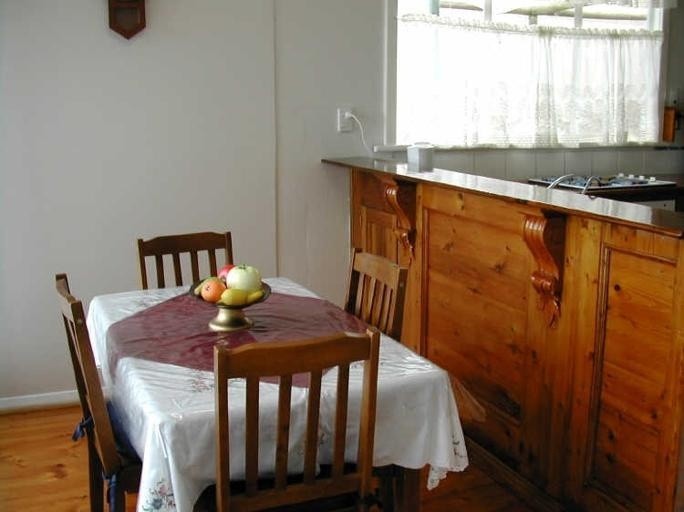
[528,173,674,189]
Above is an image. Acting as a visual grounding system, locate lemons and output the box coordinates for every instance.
[221,288,251,305]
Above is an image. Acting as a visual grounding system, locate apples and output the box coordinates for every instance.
[218,264,236,289]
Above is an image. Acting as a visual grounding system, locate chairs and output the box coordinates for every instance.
[212,326,380,512]
[341,247,408,342]
[135,231,233,289]
[54,273,140,512]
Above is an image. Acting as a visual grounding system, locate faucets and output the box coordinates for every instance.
[549,174,575,189]
[580,176,602,194]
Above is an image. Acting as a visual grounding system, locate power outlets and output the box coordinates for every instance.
[337,108,353,132]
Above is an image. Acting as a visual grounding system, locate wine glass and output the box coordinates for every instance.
[189,277,271,331]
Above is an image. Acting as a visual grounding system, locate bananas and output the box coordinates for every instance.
[218,289,266,306]
